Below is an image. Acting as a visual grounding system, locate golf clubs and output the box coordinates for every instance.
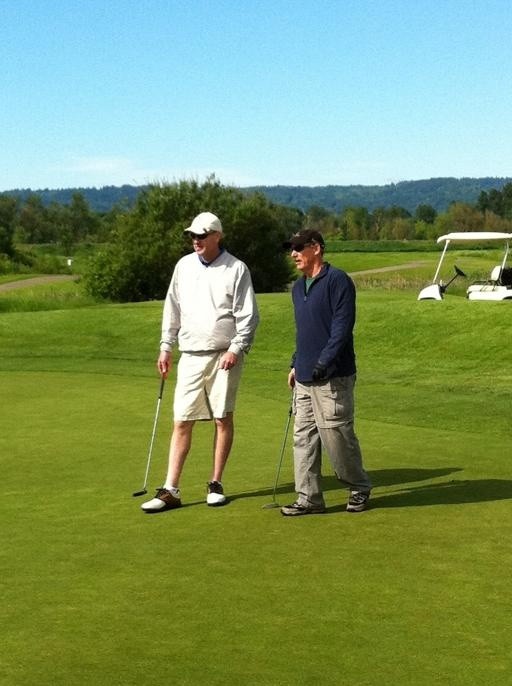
[261,381,295,509]
[133,361,168,496]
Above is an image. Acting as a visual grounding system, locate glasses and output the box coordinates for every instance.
[190,232,209,239]
[291,243,311,252]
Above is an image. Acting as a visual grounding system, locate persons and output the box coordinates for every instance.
[137,211,262,515]
[278,228,372,517]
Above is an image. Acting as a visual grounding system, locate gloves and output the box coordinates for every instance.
[311,363,328,384]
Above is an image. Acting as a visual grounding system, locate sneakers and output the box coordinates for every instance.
[346,491,369,512]
[281,501,326,516]
[206,480,226,505]
[141,486,182,512]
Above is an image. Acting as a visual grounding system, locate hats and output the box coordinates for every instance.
[183,211,223,235]
[282,229,325,249]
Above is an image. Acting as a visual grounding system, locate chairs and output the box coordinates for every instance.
[466,266,502,293]
